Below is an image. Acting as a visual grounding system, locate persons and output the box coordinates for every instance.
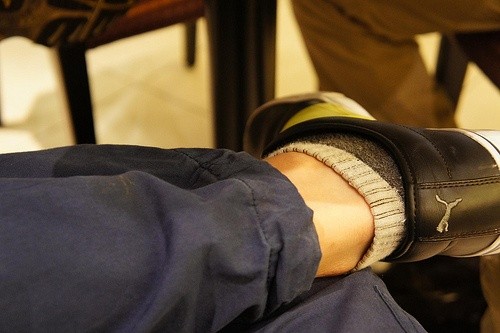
[0,92,500,333]
[290,0,500,129]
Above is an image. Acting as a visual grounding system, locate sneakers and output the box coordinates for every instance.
[374,256,490,333]
[241,89,500,264]
